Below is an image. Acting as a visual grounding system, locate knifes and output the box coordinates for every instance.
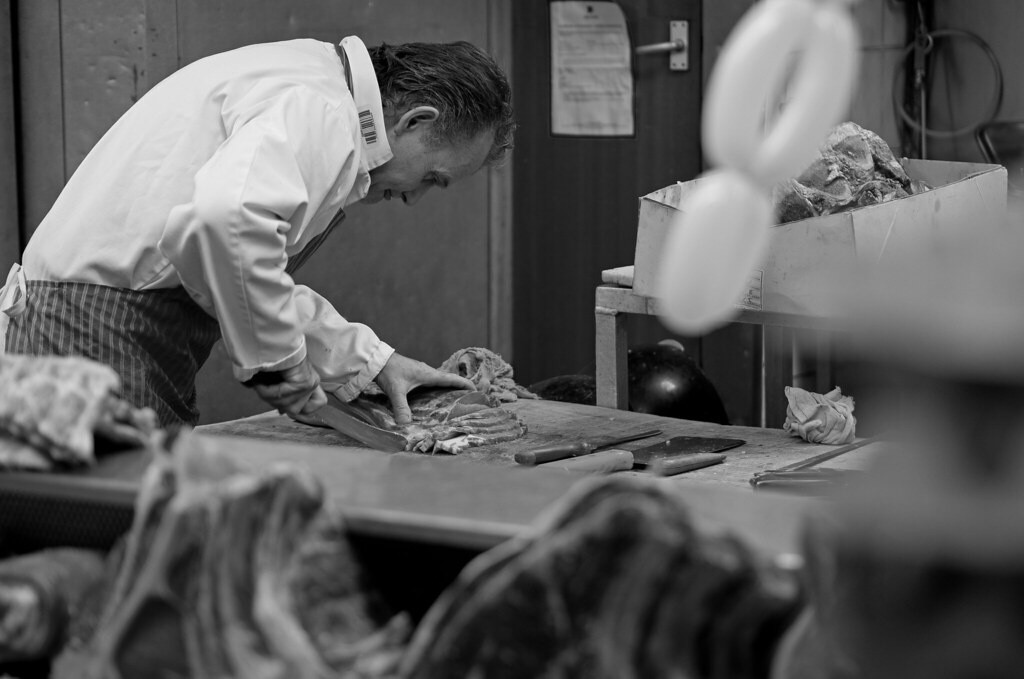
[514,431,662,465]
[564,435,747,477]
[252,369,409,453]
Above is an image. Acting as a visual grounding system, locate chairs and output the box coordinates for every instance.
[974,120,1024,198]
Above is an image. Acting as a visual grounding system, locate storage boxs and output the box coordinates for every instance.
[633,159,1009,316]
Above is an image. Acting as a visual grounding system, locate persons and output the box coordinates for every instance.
[0,33,519,459]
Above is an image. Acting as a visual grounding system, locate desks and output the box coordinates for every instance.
[596,284,861,429]
[0,401,901,609]
